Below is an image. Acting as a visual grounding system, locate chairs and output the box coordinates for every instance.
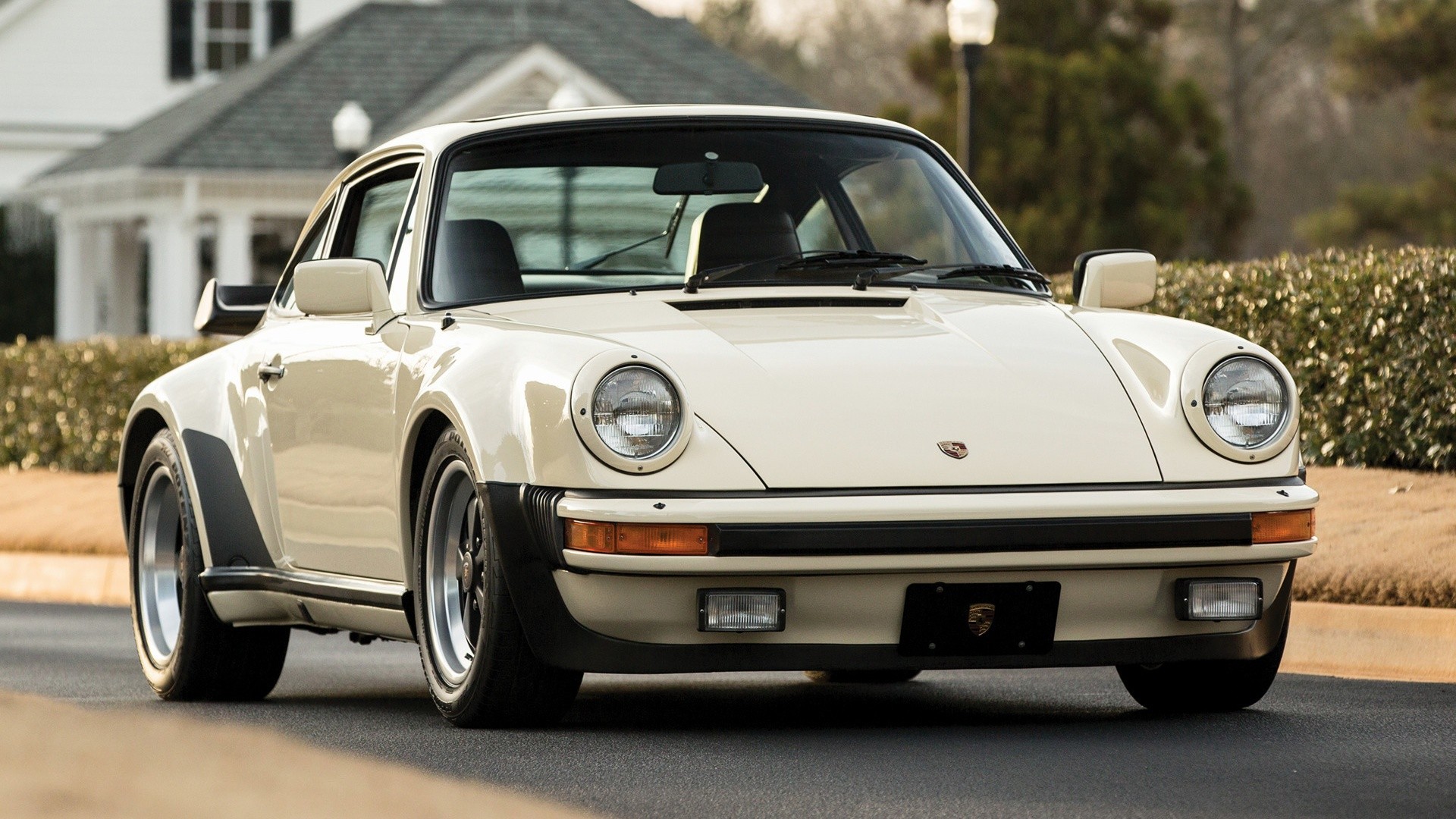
[680,201,805,281]
[428,216,526,302]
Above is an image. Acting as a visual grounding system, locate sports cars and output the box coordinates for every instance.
[113,101,1325,726]
